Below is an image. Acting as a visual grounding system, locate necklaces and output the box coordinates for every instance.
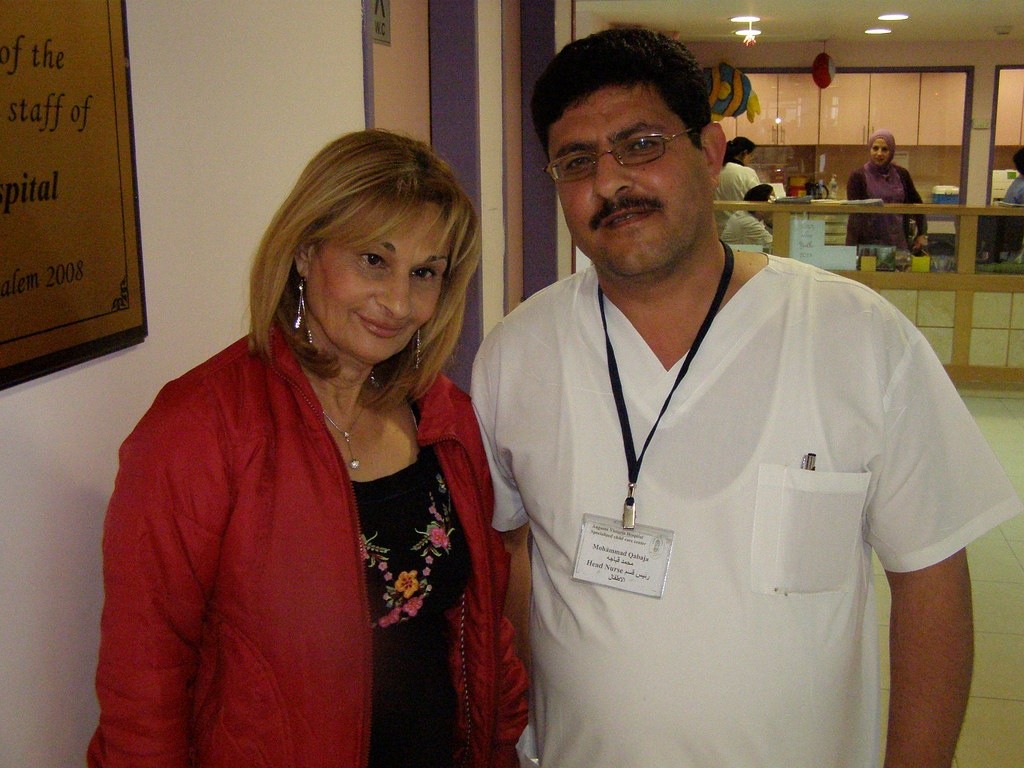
[325,399,366,469]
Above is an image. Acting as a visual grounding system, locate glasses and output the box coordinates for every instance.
[542,126,694,184]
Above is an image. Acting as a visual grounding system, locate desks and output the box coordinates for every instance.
[915,220,955,237]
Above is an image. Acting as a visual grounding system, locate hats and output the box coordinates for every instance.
[743,183,775,204]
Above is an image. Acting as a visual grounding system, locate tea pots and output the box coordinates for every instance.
[805,178,829,199]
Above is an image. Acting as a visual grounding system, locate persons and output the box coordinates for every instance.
[845,129,929,254]
[87,130,529,768]
[713,136,760,239]
[469,28,1021,768]
[1000,147,1024,207]
[718,184,773,245]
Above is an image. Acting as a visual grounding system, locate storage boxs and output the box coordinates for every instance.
[932,186,959,205]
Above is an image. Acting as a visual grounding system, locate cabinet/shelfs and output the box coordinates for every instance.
[994,69,1024,145]
[713,116,736,141]
[819,72,921,145]
[737,73,819,145]
[918,72,967,146]
